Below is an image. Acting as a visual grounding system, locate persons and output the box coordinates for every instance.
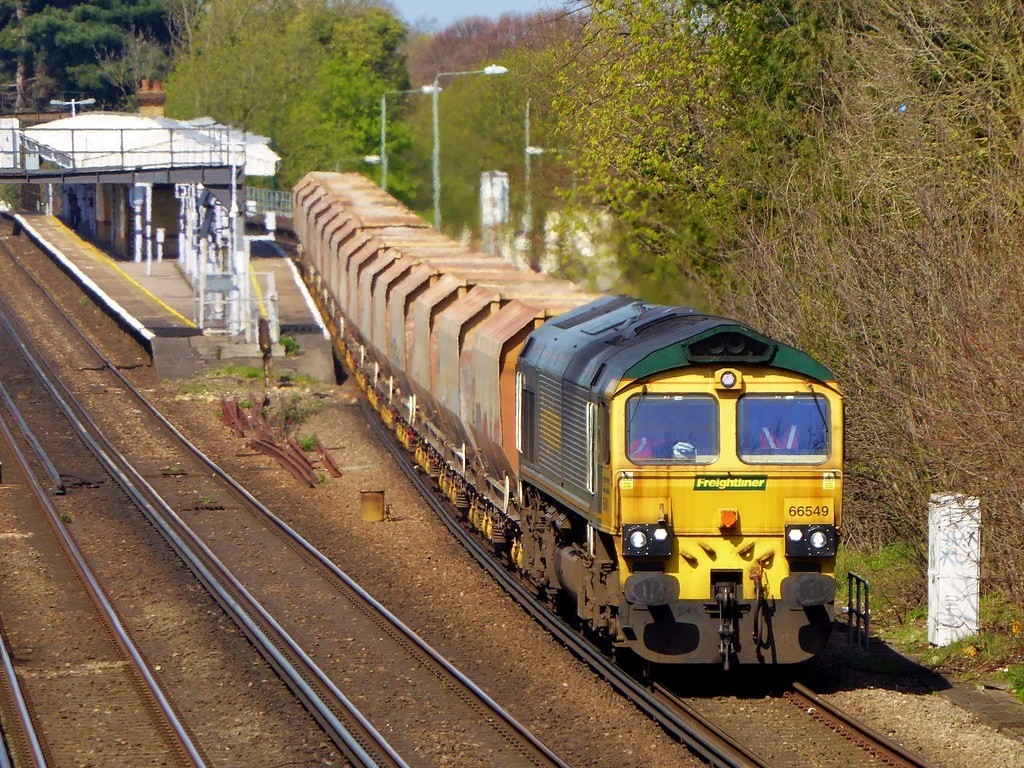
[748,408,807,448]
[67,186,81,232]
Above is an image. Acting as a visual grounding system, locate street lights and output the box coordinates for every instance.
[379,84,443,192]
[433,63,509,229]
[524,146,580,191]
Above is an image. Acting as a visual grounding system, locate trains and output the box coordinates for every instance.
[291,167,846,683]
